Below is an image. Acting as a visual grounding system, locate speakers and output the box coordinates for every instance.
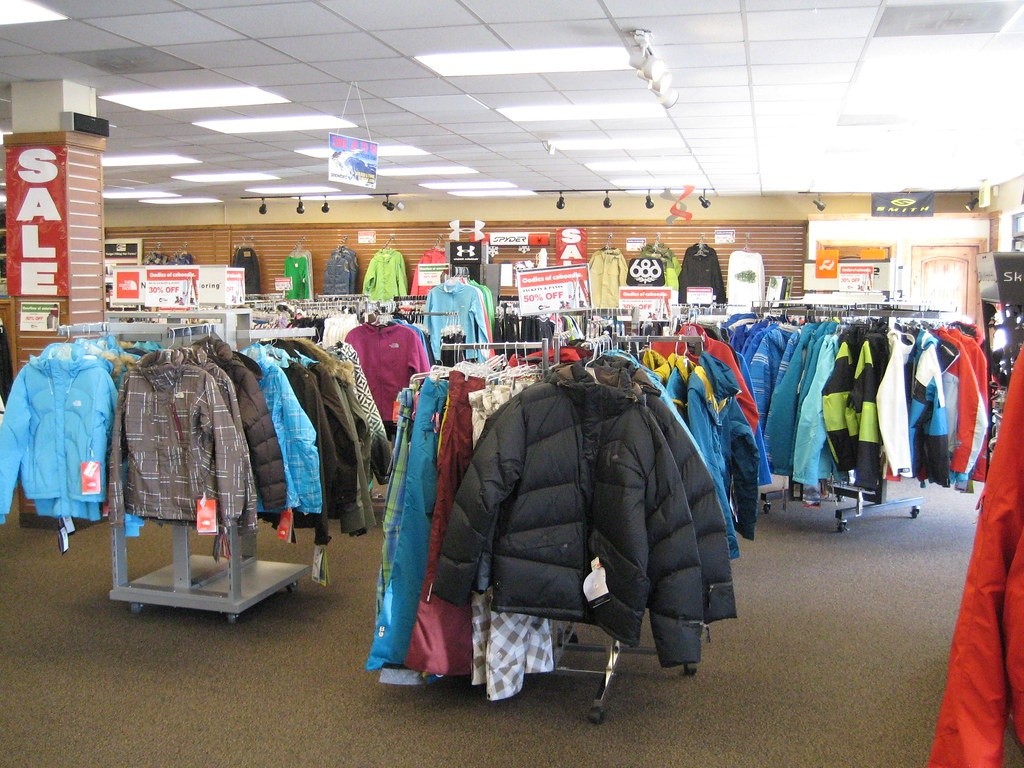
[61,112,110,138]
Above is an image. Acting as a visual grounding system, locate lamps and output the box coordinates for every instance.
[259,197,268,214]
[603,191,612,208]
[644,189,654,209]
[321,195,330,213]
[382,194,394,211]
[698,189,711,208]
[964,192,979,212]
[296,197,305,214]
[813,193,827,210]
[629,42,679,108]
[556,191,565,209]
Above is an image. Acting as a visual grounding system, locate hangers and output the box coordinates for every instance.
[50,231,979,387]
